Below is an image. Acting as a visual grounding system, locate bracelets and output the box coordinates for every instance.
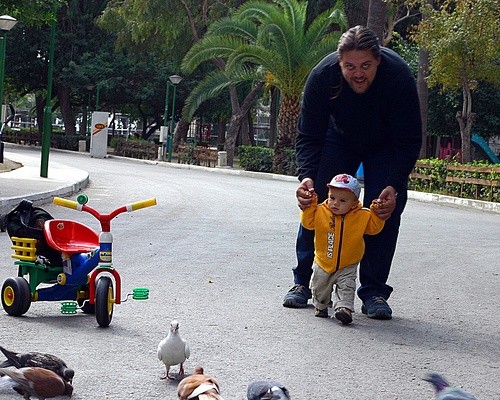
[395,190,398,199]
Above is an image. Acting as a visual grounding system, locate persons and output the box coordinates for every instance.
[283,25,422,321]
[300,174,386,324]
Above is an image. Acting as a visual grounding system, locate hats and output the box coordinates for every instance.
[327,174,361,200]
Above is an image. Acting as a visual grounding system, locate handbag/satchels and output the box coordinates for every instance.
[0,199,63,266]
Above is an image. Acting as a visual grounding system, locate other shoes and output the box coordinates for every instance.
[335,308,353,324]
[314,308,328,318]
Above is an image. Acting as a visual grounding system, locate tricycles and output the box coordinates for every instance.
[0,194,158,328]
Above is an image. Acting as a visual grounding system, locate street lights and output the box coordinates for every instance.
[87,84,96,137]
[167,74,182,162]
[0,14,18,164]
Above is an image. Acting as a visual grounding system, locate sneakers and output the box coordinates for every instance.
[361,296,393,320]
[282,284,312,308]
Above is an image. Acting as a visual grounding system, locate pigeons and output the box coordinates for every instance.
[156,320,223,400]
[421,372,477,400]
[0,346,75,400]
[246,380,291,400]
